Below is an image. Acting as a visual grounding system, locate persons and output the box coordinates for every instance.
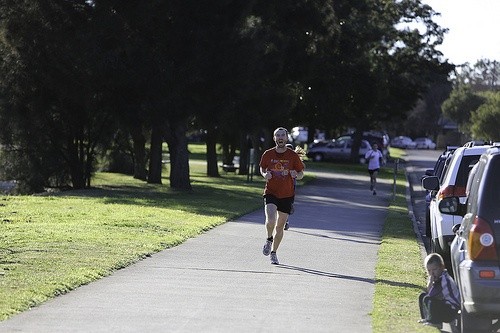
[366,143,384,194]
[417,254,460,329]
[259,127,305,264]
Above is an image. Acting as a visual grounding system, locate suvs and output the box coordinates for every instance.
[438,142,500,333]
[420,140,495,281]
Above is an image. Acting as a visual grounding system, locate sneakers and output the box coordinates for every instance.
[270,252,279,264]
[263,240,273,256]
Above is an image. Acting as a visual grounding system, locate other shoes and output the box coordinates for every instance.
[373,190,376,195]
[419,319,442,329]
[370,184,373,190]
[284,221,289,230]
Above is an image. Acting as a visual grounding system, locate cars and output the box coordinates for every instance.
[305,136,373,165]
[309,131,390,154]
[390,136,417,150]
[414,137,437,150]
